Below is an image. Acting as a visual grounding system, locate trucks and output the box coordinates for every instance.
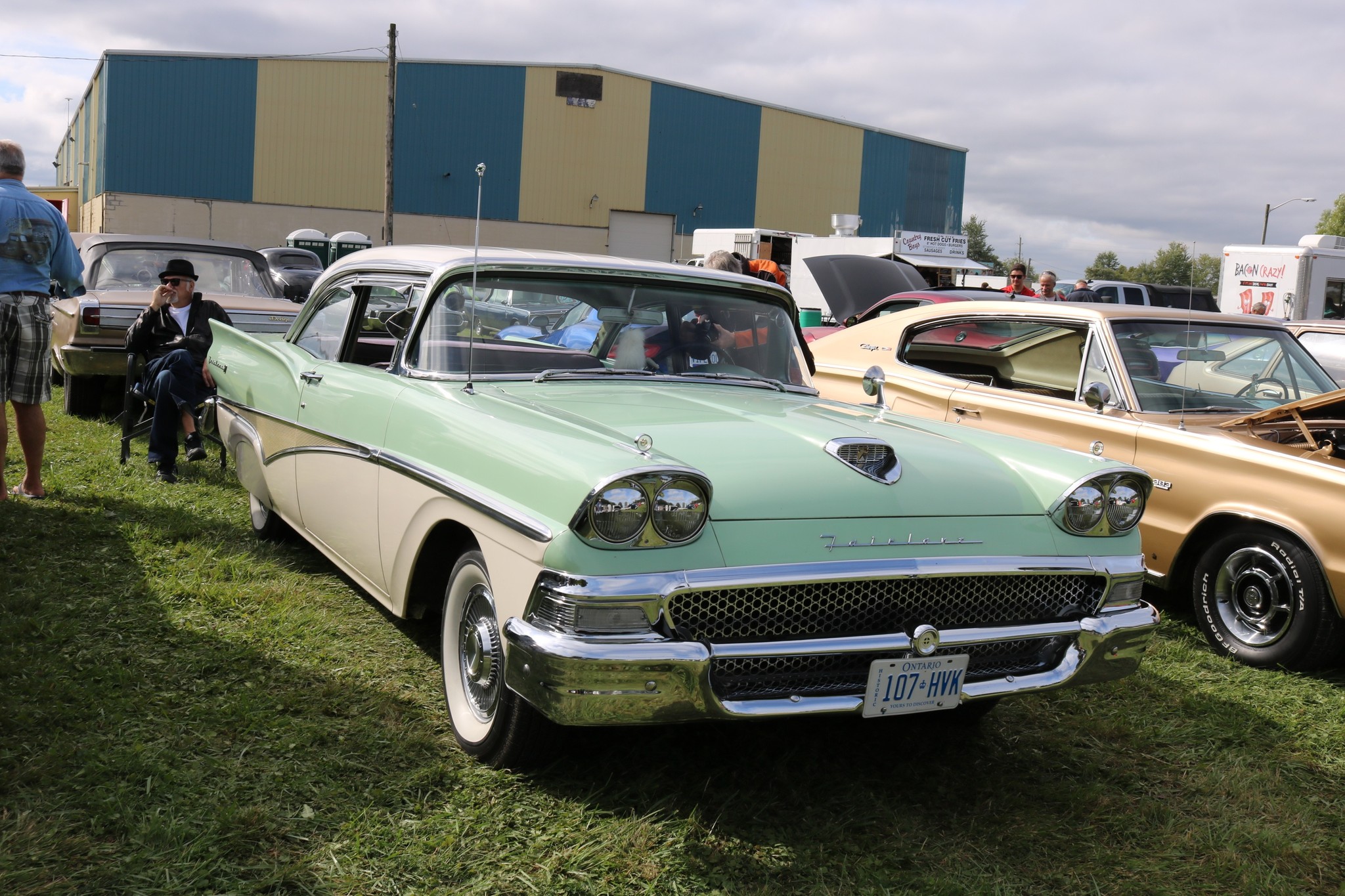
[683,228,817,312]
[1216,235,1345,367]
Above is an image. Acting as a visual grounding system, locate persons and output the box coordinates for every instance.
[940,283,947,287]
[969,269,994,276]
[998,263,1036,297]
[957,271,959,275]
[0,141,87,502]
[1031,269,1067,301]
[1248,302,1266,316]
[1065,279,1103,302]
[948,283,956,286]
[124,258,234,483]
[1029,288,1035,292]
[981,282,989,288]
[1056,290,1064,297]
[696,248,815,386]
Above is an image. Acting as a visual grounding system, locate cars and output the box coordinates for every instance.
[197,244,1161,773]
[46,233,580,416]
[493,256,1345,668]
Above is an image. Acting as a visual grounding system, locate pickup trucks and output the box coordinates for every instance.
[1035,279,1220,335]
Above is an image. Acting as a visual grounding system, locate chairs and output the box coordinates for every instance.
[1011,389,1076,401]
[942,373,997,387]
[120,352,226,469]
[1138,393,1184,412]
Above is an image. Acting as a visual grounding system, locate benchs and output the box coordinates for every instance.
[349,336,602,370]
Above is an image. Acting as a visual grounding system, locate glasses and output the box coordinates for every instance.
[1011,275,1024,279]
[160,278,190,287]
[1042,271,1055,277]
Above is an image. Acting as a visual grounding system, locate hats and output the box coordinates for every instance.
[158,259,199,282]
[1076,279,1087,285]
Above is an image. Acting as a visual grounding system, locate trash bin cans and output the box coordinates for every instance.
[799,306,823,327]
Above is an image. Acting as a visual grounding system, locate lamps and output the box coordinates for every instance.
[589,194,599,208]
[69,137,75,143]
[52,162,61,168]
[693,203,703,217]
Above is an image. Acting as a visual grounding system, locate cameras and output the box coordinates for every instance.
[684,320,720,360]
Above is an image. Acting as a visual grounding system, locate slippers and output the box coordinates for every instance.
[10,482,45,498]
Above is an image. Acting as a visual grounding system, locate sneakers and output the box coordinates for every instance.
[184,431,207,462]
[154,461,179,483]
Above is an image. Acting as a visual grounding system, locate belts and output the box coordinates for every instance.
[5,290,50,300]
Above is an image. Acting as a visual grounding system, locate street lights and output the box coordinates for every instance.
[1261,198,1316,245]
